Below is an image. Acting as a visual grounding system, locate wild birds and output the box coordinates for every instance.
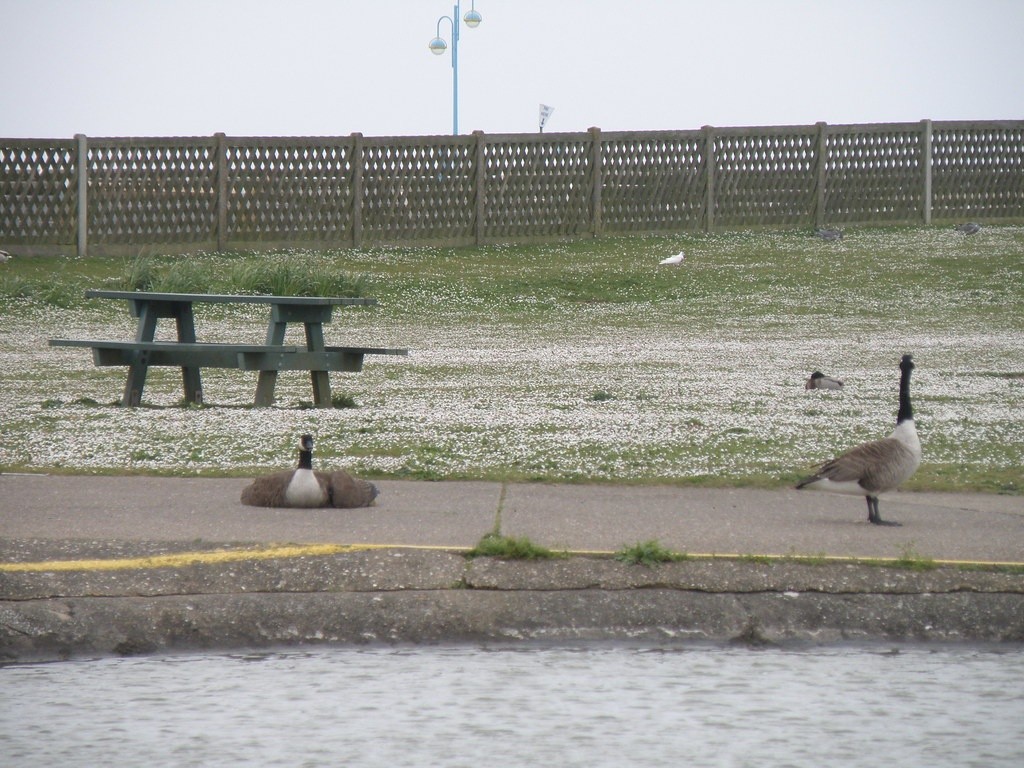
[810,228,843,241]
[658,251,686,268]
[794,351,923,527]
[0,250,13,264]
[954,221,981,236]
[240,433,382,510]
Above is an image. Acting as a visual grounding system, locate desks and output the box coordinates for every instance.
[85,290,378,408]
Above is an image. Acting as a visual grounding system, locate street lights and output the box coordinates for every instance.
[428,0,483,135]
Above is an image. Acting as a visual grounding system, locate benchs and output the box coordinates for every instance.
[48,339,297,354]
[300,346,409,356]
